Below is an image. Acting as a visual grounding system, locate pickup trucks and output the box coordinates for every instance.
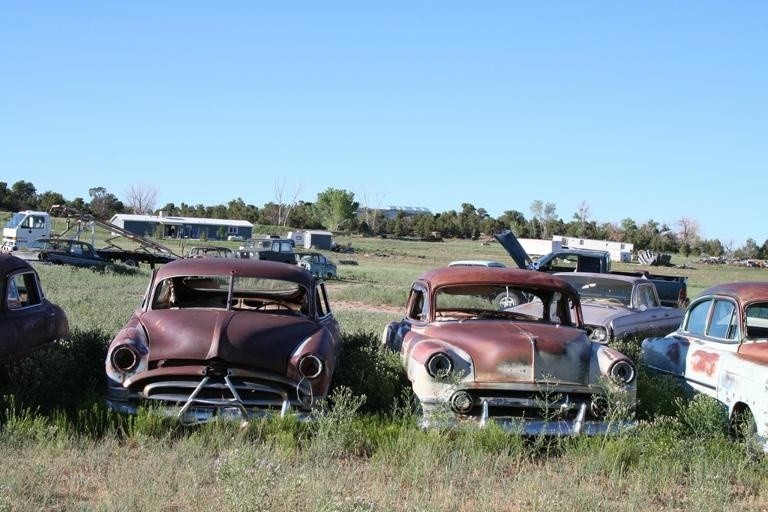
[488,228,688,313]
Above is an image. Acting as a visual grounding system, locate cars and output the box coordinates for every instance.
[186,246,236,259]
[380,265,638,443]
[27,237,110,271]
[0,250,68,365]
[297,253,336,279]
[444,261,505,269]
[103,254,344,432]
[507,271,693,348]
[638,276,767,455]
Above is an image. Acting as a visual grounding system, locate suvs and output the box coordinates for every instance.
[239,234,295,265]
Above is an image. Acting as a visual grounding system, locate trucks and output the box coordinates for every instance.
[0,203,185,270]
